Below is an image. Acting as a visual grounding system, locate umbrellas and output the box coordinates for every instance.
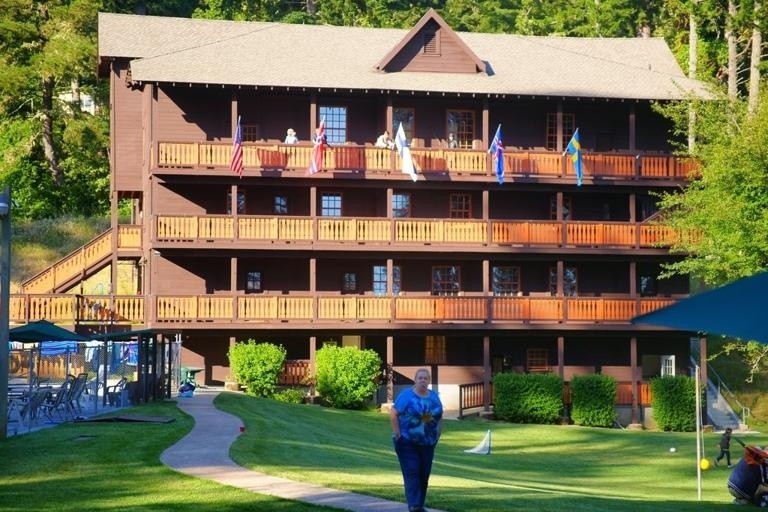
[10,320,90,342]
[628,272,768,346]
[92,327,177,344]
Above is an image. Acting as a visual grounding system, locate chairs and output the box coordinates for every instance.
[7,371,130,436]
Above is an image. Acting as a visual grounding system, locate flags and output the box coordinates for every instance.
[487,126,505,192]
[308,121,324,174]
[228,115,245,180]
[395,126,418,183]
[566,129,583,188]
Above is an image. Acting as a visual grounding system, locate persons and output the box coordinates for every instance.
[283,128,299,144]
[374,129,395,150]
[447,133,460,150]
[390,369,444,512]
[713,428,734,469]
[726,444,768,508]
